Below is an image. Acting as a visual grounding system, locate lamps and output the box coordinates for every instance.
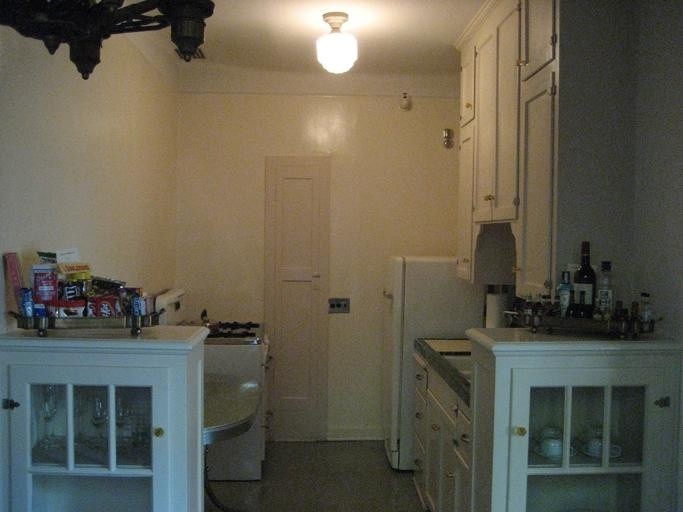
[0,0,218,84]
[315,11,361,77]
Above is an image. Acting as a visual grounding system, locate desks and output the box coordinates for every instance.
[199,372,262,511]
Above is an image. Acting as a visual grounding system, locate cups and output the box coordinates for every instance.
[534,424,623,461]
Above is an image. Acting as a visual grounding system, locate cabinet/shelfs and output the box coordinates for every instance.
[470,0,520,224]
[411,350,472,511]
[0,323,211,511]
[514,1,637,308]
[452,16,518,286]
[464,327,683,512]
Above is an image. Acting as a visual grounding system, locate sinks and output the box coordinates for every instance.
[442,352,472,375]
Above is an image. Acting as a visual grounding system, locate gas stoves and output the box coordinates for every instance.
[184,320,265,346]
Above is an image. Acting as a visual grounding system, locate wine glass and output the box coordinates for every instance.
[38,385,128,462]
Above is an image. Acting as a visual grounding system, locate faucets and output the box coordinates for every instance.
[503,309,521,320]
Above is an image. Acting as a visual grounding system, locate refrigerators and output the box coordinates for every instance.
[382,256,485,471]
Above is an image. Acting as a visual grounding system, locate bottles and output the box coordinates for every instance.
[524,240,655,342]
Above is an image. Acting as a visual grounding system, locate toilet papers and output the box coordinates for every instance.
[484,292,508,330]
